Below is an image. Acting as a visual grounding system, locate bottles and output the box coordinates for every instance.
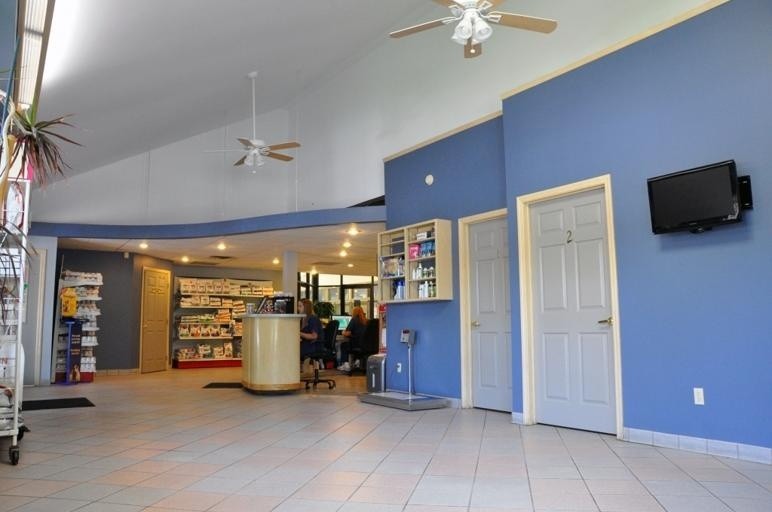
[412,262,436,299]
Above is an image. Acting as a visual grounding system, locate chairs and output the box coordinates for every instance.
[349,319,379,376]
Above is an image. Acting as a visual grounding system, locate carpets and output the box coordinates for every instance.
[202,383,243,388]
[22,398,95,411]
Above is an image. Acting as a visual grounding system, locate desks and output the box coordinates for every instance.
[335,335,349,366]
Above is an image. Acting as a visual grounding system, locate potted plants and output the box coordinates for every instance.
[313,302,336,323]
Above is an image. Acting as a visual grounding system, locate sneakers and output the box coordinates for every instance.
[337,359,360,373]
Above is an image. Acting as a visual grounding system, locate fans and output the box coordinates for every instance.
[390,1,558,58]
[201,71,301,166]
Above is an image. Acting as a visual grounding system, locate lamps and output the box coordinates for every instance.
[244,149,266,167]
[452,10,493,45]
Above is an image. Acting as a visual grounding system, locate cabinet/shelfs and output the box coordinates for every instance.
[0,177,31,465]
[379,304,386,352]
[378,219,453,303]
[306,320,339,390]
[172,276,274,369]
[51,271,104,383]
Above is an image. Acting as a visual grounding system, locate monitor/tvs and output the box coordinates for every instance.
[331,315,354,331]
[273,296,295,313]
[645,159,743,234]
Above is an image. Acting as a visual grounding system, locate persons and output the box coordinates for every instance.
[298,298,324,361]
[337,305,369,372]
